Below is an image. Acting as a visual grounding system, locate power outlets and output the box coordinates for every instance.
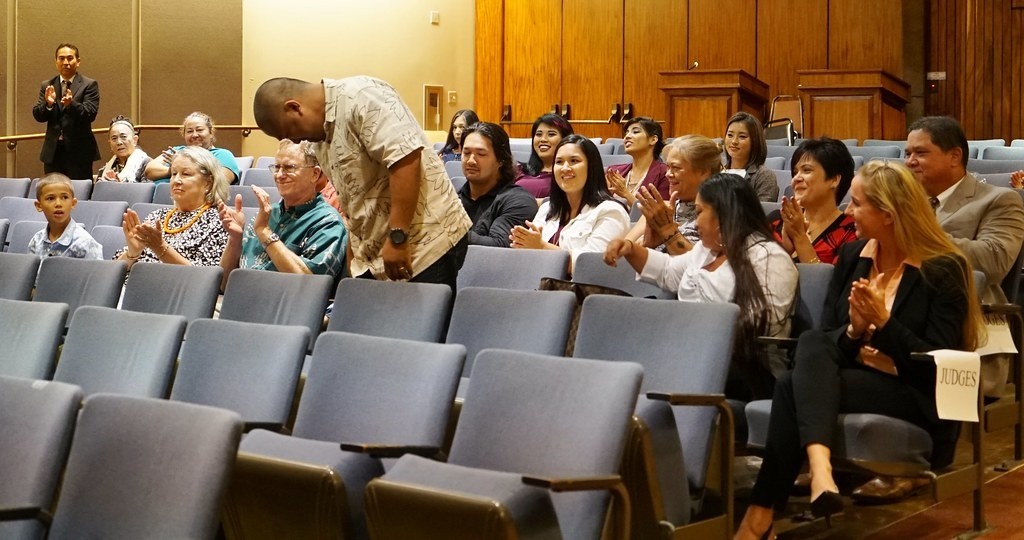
[448,91,457,104]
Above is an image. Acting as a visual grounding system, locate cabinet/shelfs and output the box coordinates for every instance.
[473,0,926,141]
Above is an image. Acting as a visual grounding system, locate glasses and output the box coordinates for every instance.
[268,164,313,175]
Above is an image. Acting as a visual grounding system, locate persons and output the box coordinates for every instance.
[766,137,859,267]
[112,145,229,309]
[509,134,630,274]
[253,75,472,294]
[457,121,539,247]
[513,113,575,197]
[33,43,101,179]
[27,173,104,286]
[604,117,671,205]
[603,173,799,402]
[904,116,1024,466]
[635,134,723,256]
[216,138,348,291]
[94,115,153,183]
[723,112,780,203]
[145,112,241,185]
[438,109,479,163]
[733,159,989,540]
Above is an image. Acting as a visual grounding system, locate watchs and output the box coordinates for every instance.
[389,228,410,245]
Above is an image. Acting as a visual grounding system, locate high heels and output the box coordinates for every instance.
[811,488,843,528]
[759,523,778,540]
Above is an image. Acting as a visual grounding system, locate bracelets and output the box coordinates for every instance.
[629,240,633,247]
[809,256,820,264]
[262,233,279,249]
[158,244,169,258]
[126,251,139,260]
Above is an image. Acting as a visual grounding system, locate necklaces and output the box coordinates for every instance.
[164,204,211,234]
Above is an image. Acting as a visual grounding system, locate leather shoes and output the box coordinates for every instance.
[850,473,930,503]
[793,462,812,493]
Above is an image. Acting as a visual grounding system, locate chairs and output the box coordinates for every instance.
[0,138,1024,540]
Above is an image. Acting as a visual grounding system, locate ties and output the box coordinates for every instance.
[59,80,71,141]
[928,197,939,216]
